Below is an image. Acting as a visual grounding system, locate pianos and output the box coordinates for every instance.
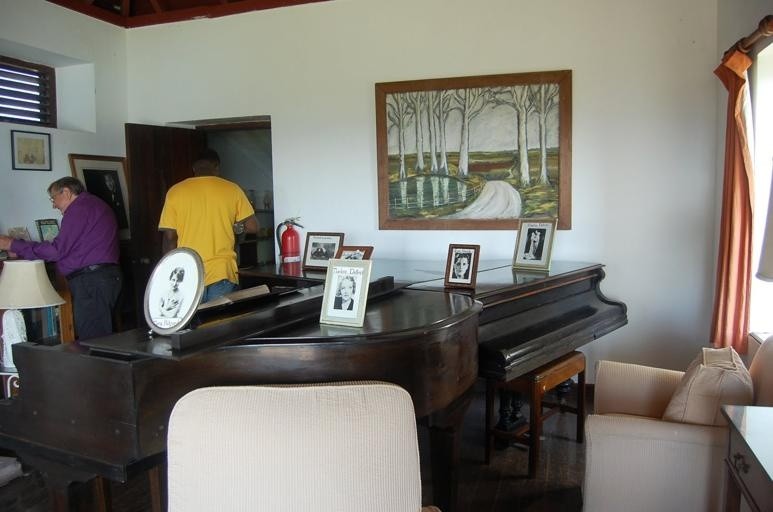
[236,255,628,469]
[0,275,485,512]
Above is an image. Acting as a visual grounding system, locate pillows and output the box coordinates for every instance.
[655,346,756,427]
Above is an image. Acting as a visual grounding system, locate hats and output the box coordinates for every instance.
[191,147,220,162]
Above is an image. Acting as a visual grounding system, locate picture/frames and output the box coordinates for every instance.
[332,242,375,260]
[317,256,373,328]
[298,230,345,273]
[8,130,51,172]
[441,241,480,293]
[509,216,559,275]
[65,152,136,245]
[140,245,206,338]
[371,68,574,234]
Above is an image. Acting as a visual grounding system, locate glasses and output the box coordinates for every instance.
[48,187,64,202]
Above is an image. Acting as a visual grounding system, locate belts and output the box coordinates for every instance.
[64,263,118,281]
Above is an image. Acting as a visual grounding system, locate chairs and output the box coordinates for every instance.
[164,379,423,512]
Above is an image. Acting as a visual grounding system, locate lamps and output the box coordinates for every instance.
[0,256,67,344]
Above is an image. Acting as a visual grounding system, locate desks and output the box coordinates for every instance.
[0,361,21,399]
[714,404,772,512]
[235,264,325,291]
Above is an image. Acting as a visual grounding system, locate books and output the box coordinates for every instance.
[31,307,57,339]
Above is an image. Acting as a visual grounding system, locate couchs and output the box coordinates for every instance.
[579,326,771,512]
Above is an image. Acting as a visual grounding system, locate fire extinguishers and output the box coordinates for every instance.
[276,217,304,277]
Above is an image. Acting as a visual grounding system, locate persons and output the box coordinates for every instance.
[452,253,470,279]
[0,177,124,340]
[157,147,260,304]
[158,267,185,319]
[334,276,356,310]
[104,174,127,229]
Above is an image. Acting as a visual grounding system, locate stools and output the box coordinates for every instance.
[479,348,587,477]
